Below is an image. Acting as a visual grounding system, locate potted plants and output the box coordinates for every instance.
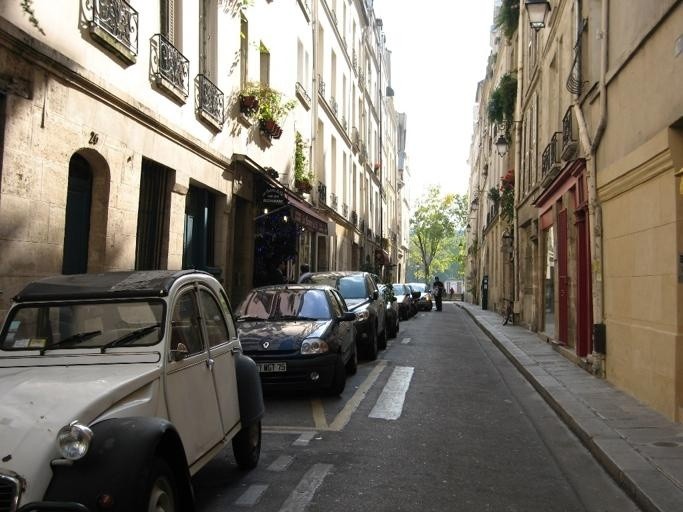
[239,84,298,139]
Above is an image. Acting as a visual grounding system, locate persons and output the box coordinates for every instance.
[433,276,446,312]
